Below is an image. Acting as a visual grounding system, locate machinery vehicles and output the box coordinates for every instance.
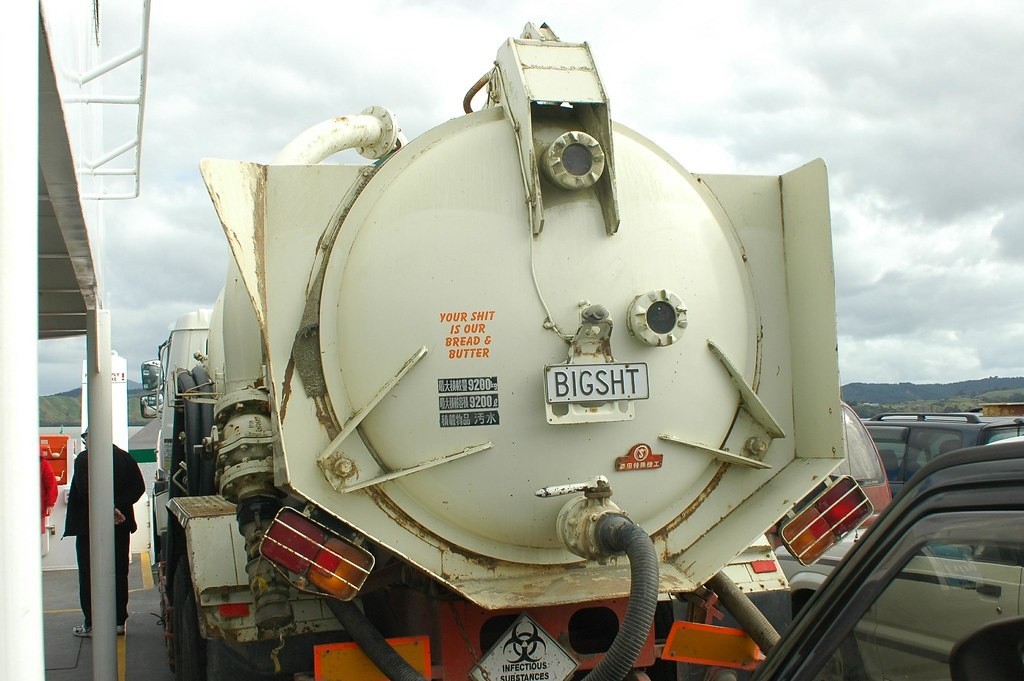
[140,21,877,681]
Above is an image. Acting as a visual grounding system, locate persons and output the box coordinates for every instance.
[60,426,146,636]
[40,446,58,557]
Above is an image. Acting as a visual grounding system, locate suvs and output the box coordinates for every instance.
[750,400,1024,681]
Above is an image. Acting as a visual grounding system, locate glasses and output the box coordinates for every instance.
[82,439,87,444]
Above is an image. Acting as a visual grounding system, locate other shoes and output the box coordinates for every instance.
[117,625,124,635]
[72,624,92,637]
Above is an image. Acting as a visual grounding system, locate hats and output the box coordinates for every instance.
[81,427,88,439]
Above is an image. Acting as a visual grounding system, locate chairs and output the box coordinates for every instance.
[879,448,901,481]
[932,440,963,456]
